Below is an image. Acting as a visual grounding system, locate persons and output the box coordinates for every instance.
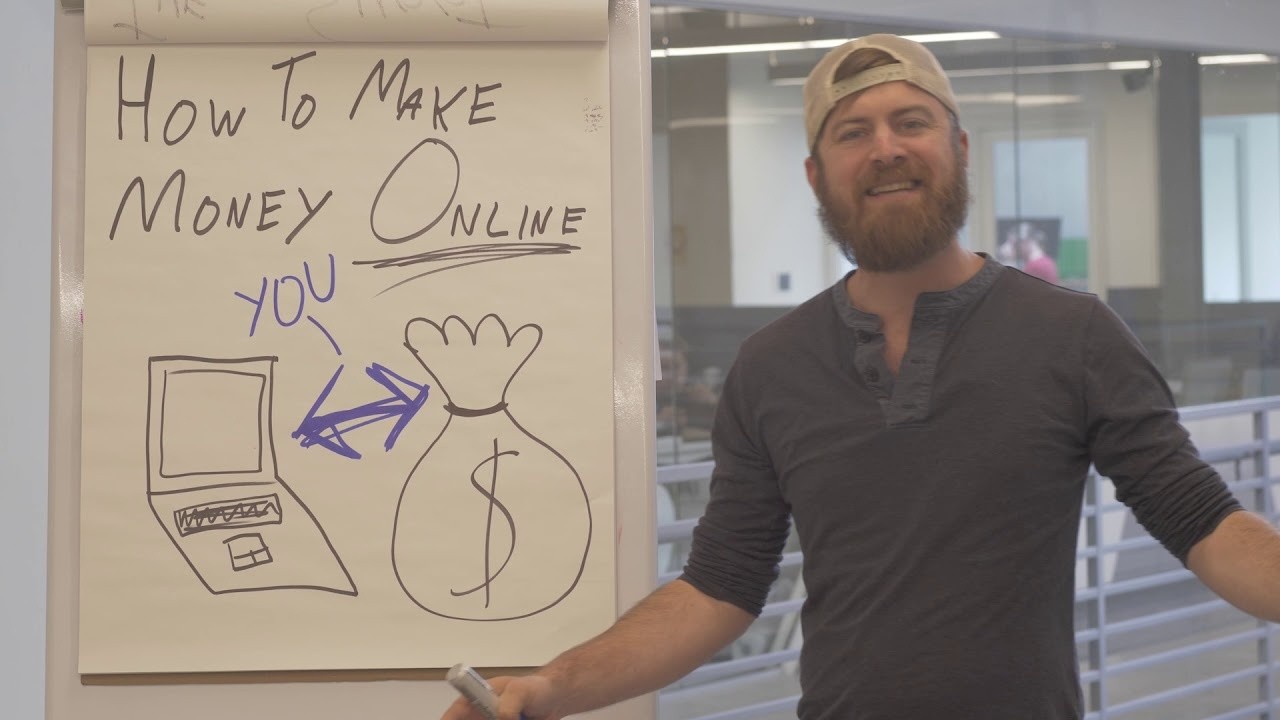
[1009,222,1059,285]
[664,347,717,427]
[441,33,1280,720]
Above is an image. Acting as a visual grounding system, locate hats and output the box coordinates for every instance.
[802,34,960,155]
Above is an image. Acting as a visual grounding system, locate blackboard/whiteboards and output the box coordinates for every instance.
[44,0,665,720]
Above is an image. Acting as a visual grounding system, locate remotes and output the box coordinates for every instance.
[447,665,526,720]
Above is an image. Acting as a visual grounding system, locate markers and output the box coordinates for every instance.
[447,662,527,719]
